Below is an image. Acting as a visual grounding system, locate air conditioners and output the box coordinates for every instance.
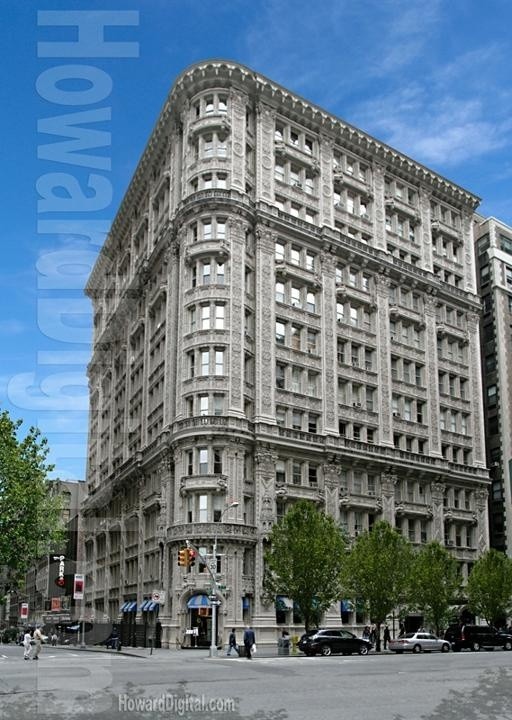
[308,348,317,355]
[338,317,345,323]
[445,540,455,546]
[293,302,302,309]
[352,402,361,408]
[394,412,400,418]
[391,336,399,341]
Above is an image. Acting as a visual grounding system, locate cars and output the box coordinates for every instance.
[299,629,373,656]
[443,623,512,651]
[389,632,452,653]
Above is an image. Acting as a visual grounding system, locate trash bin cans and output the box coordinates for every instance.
[238,646,246,657]
[278,638,289,655]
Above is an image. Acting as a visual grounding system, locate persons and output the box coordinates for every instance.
[417,626,426,633]
[226,628,239,656]
[398,624,404,637]
[23,628,34,660]
[362,626,370,637]
[52,633,58,646]
[383,625,391,650]
[243,625,255,658]
[32,624,48,660]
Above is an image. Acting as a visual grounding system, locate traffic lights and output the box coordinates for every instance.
[177,548,195,567]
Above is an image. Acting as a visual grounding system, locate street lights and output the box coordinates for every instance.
[209,502,239,657]
